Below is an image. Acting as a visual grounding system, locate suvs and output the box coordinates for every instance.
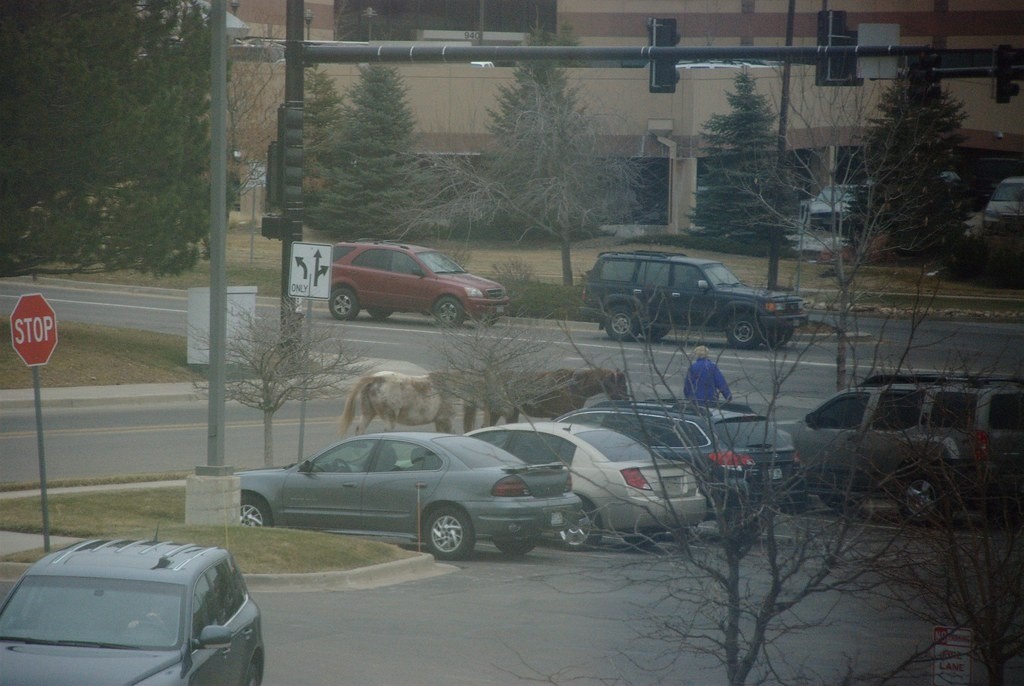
[771,374,1023,526]
[551,399,808,544]
[578,248,810,350]
[1,522,265,686]
[328,235,510,329]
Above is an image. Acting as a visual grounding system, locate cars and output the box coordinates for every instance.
[231,430,583,561]
[808,183,859,235]
[394,421,705,550]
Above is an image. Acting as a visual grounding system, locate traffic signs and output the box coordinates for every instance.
[287,240,331,302]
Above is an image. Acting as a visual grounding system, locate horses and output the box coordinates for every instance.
[464,367,629,435]
[337,370,514,442]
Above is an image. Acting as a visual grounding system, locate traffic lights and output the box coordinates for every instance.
[994,45,1020,104]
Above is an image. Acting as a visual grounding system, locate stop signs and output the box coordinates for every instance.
[11,292,59,367]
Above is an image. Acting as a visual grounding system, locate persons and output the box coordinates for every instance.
[683,346,733,402]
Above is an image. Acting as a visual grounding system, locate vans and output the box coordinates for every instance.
[982,176,1024,237]
